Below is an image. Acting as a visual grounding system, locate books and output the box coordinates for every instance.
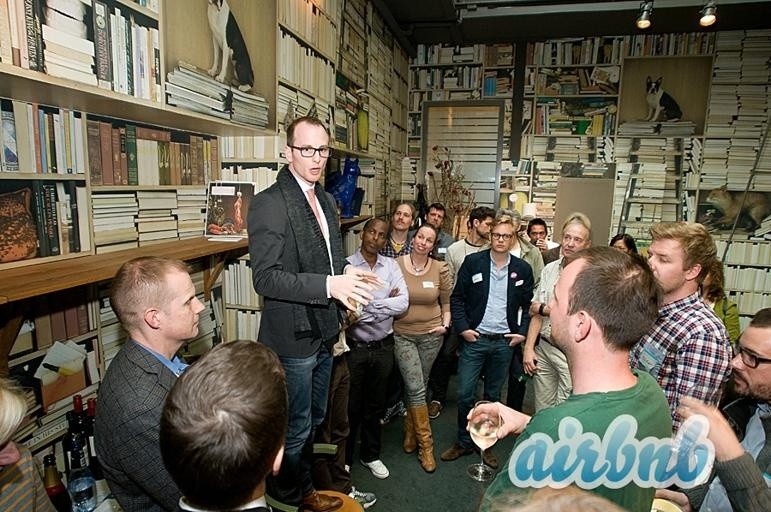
[1,0,771,512]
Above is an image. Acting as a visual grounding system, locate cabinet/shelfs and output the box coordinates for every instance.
[1,0,770,512]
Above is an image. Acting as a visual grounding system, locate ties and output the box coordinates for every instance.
[306,188,322,231]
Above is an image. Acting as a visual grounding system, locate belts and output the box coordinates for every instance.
[481,334,503,339]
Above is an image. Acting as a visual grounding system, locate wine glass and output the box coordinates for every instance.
[465,400,501,483]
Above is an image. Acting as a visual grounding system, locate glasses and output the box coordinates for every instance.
[288,145,334,158]
[491,233,514,241]
[734,339,771,369]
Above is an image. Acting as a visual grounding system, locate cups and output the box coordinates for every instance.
[676,383,722,434]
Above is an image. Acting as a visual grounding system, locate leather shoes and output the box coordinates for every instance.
[304,490,343,512]
[476,445,498,469]
[441,443,473,462]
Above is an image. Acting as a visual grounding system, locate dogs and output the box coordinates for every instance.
[205,0,255,94]
[644,75,683,123]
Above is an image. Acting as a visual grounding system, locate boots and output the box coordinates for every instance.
[403,406,417,453]
[410,403,436,472]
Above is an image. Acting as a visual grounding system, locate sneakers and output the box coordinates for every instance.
[380,400,408,425]
[427,400,443,419]
[348,486,377,509]
[359,459,390,480]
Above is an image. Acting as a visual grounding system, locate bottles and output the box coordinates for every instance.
[42,394,102,512]
